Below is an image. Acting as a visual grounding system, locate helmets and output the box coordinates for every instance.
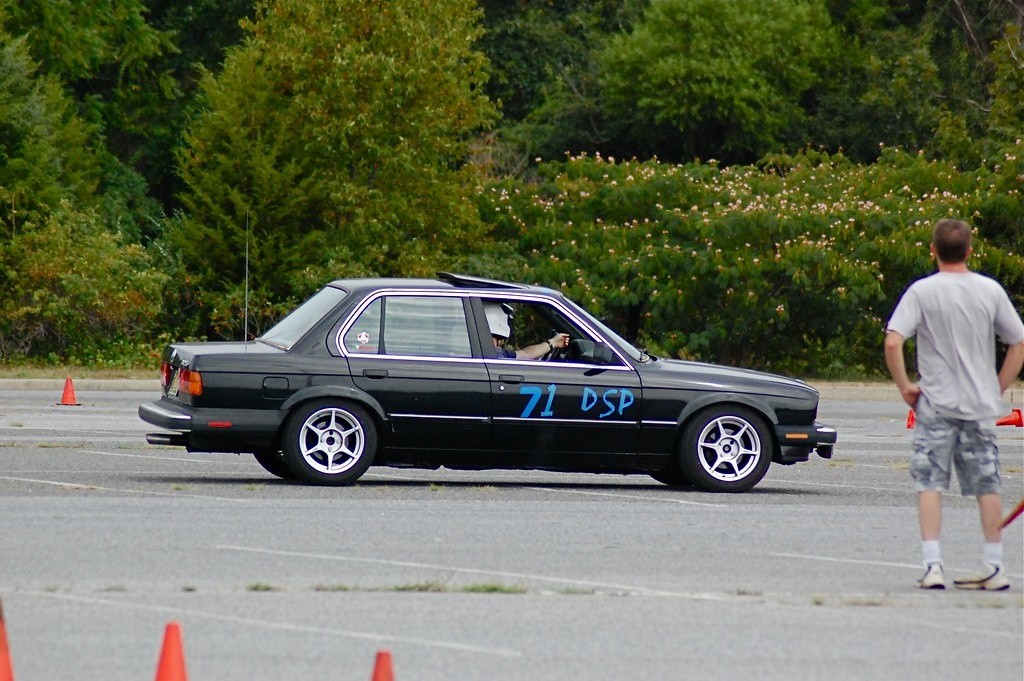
[483,302,514,340]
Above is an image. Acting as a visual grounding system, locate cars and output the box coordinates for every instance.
[138,270,838,492]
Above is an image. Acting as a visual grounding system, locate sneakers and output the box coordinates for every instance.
[953,560,1010,591]
[915,559,946,589]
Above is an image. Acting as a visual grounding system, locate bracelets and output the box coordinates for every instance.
[545,340,554,352]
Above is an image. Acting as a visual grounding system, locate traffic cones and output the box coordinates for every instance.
[57,376,82,406]
[368,651,397,681]
[993,407,1023,427]
[906,409,917,427]
[153,621,186,681]
[0,600,15,681]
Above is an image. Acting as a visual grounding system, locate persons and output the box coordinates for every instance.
[484,303,569,360]
[885,219,1024,592]
